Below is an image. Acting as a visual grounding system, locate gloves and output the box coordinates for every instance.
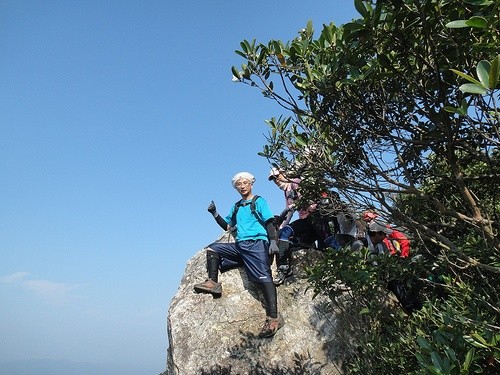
[268,240,279,254]
[208,200,218,218]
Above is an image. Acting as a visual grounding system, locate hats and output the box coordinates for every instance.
[268,166,285,181]
[363,210,378,220]
[231,171,256,188]
[369,221,393,234]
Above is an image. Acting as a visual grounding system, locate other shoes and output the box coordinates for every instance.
[272,265,293,284]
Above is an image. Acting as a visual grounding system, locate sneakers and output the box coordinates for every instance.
[259,313,285,338]
[194,278,222,294]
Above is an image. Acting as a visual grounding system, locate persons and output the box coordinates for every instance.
[268,167,409,286]
[195,173,285,337]
